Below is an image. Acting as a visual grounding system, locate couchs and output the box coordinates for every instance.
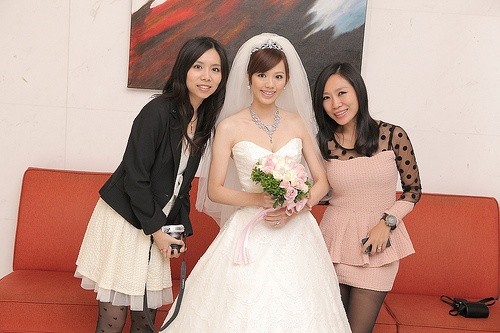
[0,168,500,333]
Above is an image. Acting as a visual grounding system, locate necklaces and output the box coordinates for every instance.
[251,104,280,143]
[190,116,198,133]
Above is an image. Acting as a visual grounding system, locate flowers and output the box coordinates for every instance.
[252,154,310,216]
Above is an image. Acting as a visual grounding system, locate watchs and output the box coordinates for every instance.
[379,213,398,230]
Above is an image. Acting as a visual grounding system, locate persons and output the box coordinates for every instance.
[314,62,422,333]
[160,32,354,333]
[73,37,229,333]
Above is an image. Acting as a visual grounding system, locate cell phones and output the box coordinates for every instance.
[360,236,392,253]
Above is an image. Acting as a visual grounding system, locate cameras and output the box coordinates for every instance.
[161,224,185,253]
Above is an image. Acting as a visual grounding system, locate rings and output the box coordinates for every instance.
[276,221,279,225]
[378,248,381,250]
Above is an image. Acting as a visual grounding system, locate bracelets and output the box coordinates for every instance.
[305,204,313,211]
